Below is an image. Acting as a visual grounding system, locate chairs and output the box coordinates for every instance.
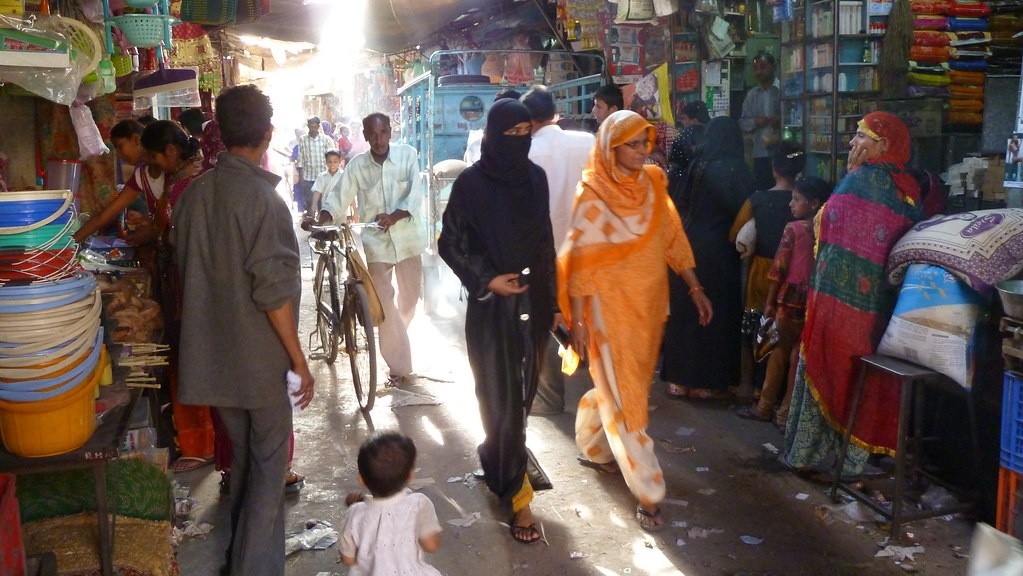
[830,353,999,544]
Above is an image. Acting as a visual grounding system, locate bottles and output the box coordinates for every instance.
[95,346,114,398]
[96,58,116,93]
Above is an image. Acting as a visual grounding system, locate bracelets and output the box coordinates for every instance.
[688,286,705,295]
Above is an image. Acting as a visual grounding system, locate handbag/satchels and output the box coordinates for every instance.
[342,223,385,327]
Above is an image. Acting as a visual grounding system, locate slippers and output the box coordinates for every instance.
[171,454,217,473]
[577,452,622,475]
[285,471,304,491]
[511,516,540,544]
[636,499,664,531]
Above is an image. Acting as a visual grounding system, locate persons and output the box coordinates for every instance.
[557,109,714,532]
[337,432,444,576]
[435,98,561,543]
[72,106,426,492]
[1009,134,1023,175]
[169,85,315,576]
[494,47,832,430]
[776,112,926,482]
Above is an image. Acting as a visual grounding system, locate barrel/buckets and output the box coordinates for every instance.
[0,189,105,458]
[48,158,83,217]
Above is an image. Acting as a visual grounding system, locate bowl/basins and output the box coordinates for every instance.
[995,279,1022,320]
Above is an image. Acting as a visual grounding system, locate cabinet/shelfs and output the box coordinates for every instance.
[780,0,886,191]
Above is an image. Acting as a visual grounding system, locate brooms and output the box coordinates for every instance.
[133,1,198,99]
[168,1,217,66]
[112,48,151,102]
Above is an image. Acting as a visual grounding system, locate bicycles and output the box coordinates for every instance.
[300,209,391,413]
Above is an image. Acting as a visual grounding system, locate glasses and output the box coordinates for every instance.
[624,140,652,149]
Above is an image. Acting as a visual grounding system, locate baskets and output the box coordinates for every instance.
[37,17,101,76]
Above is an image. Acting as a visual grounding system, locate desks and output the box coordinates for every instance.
[0,326,164,576]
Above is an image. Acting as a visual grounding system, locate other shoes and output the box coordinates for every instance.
[531,402,564,414]
[736,408,769,422]
[667,382,684,397]
[687,387,714,401]
